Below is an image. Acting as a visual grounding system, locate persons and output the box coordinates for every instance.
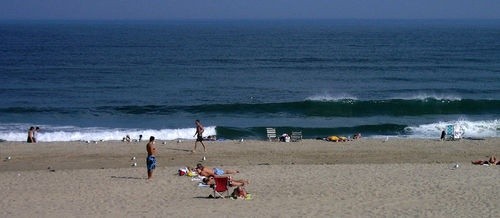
[146,136,156,179]
[26,125,40,143]
[279,132,287,139]
[471,155,500,165]
[191,120,206,152]
[196,163,245,187]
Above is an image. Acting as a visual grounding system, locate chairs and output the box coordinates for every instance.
[266,128,280,142]
[212,176,230,200]
[291,130,303,142]
[441,125,454,142]
